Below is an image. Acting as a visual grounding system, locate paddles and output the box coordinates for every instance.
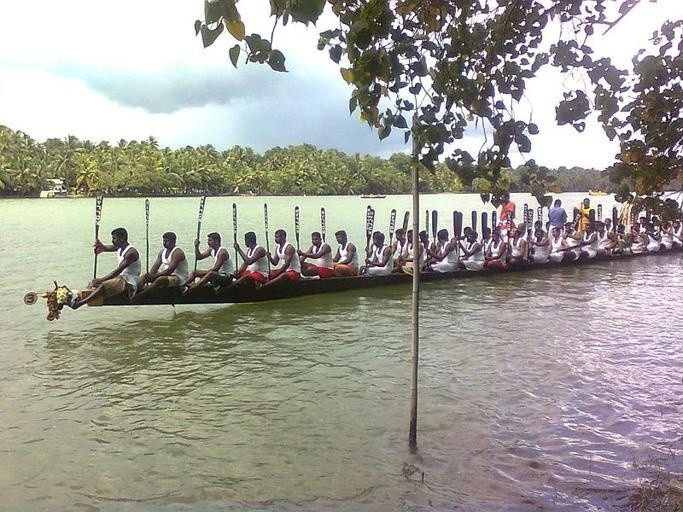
[432,210,437,244]
[94,195,103,278]
[232,203,237,274]
[389,209,396,245]
[567,207,581,238]
[472,211,476,230]
[402,211,409,236]
[598,204,602,220]
[453,211,462,255]
[321,208,325,242]
[524,204,533,256]
[295,206,299,250]
[507,212,512,250]
[145,199,150,286]
[492,211,496,231]
[482,213,487,255]
[612,207,617,232]
[537,207,542,221]
[589,209,595,233]
[426,210,429,232]
[366,206,375,266]
[264,203,270,274]
[194,195,206,282]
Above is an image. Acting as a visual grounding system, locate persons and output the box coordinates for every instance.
[233,231,270,285]
[185,232,233,290]
[496,191,516,235]
[135,232,189,298]
[359,221,550,277]
[262,229,302,288]
[66,227,141,310]
[619,194,639,235]
[296,231,333,278]
[577,198,595,234]
[332,229,359,277]
[550,208,683,262]
[548,199,568,238]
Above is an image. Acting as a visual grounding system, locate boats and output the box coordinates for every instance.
[40,190,68,198]
[591,191,607,196]
[41,247,681,321]
[361,196,386,199]
